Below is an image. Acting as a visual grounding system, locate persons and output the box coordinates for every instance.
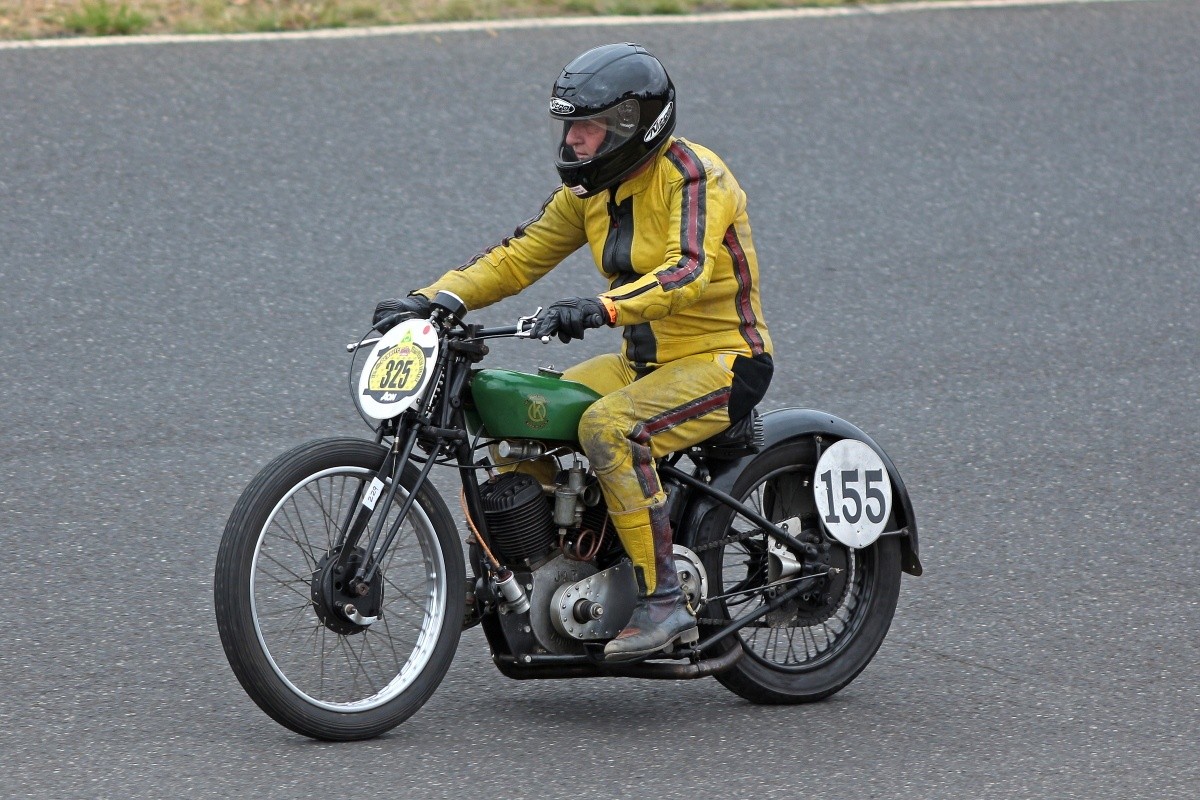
[371,44,774,662]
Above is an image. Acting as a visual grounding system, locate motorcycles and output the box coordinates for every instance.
[213,289,925,745]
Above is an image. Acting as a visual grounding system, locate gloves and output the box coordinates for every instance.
[530,296,605,344]
[372,294,431,335]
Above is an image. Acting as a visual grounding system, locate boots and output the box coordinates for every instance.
[604,494,699,660]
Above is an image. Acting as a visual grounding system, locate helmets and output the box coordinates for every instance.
[548,41,676,199]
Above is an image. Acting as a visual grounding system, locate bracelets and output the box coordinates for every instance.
[596,296,616,324]
